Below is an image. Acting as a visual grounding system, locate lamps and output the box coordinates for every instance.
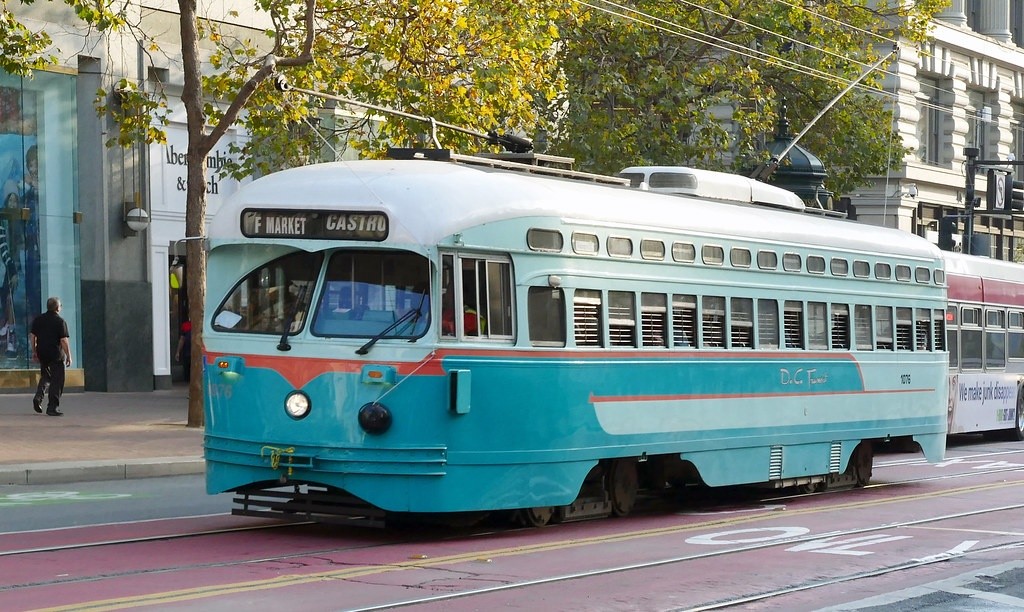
[123,201,150,236]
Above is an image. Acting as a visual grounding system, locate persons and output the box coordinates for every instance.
[443,279,485,337]
[0,179,27,360]
[22,144,43,363]
[175,307,190,399]
[29,297,72,417]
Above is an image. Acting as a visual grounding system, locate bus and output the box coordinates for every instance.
[168,146,952,532]
[936,250,1023,447]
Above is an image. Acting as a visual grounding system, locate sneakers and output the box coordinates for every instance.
[33,398,44,414]
[46,409,64,417]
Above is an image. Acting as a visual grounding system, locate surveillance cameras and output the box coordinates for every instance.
[909,186,918,197]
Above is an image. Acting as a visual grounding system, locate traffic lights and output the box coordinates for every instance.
[940,217,956,253]
